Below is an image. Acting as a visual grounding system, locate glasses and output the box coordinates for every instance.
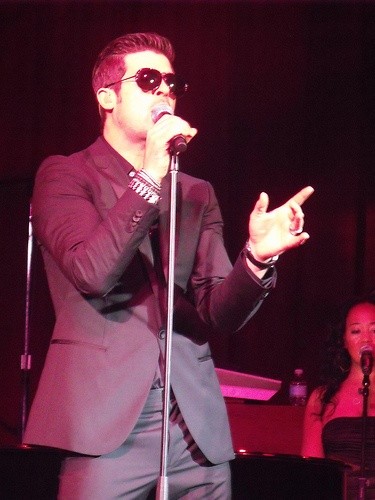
[103,68,179,94]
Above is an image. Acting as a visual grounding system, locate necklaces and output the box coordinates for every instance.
[347,377,364,406]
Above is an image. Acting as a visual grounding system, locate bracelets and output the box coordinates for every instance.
[244,241,279,268]
[139,168,161,189]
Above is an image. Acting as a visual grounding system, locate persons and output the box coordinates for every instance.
[23,33,314,500]
[300,296,375,500]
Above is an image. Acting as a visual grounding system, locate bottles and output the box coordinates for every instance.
[289,369,308,406]
